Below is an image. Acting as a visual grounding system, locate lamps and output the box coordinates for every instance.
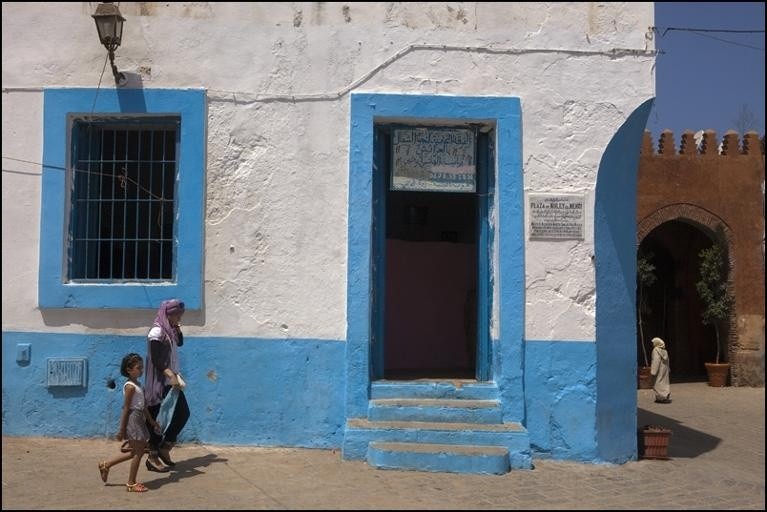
[91,3,129,87]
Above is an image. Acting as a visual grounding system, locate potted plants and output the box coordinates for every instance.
[637,258,656,390]
[695,224,735,388]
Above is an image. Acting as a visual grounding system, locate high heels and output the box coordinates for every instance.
[145,459,170,472]
[161,456,177,467]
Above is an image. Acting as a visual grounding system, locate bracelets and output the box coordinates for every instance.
[176,326,181,328]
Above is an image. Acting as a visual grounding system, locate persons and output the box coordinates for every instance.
[650,337,672,404]
[143,298,191,474]
[98,352,162,493]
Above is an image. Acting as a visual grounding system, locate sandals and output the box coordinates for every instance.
[98,461,110,481]
[126,482,148,493]
